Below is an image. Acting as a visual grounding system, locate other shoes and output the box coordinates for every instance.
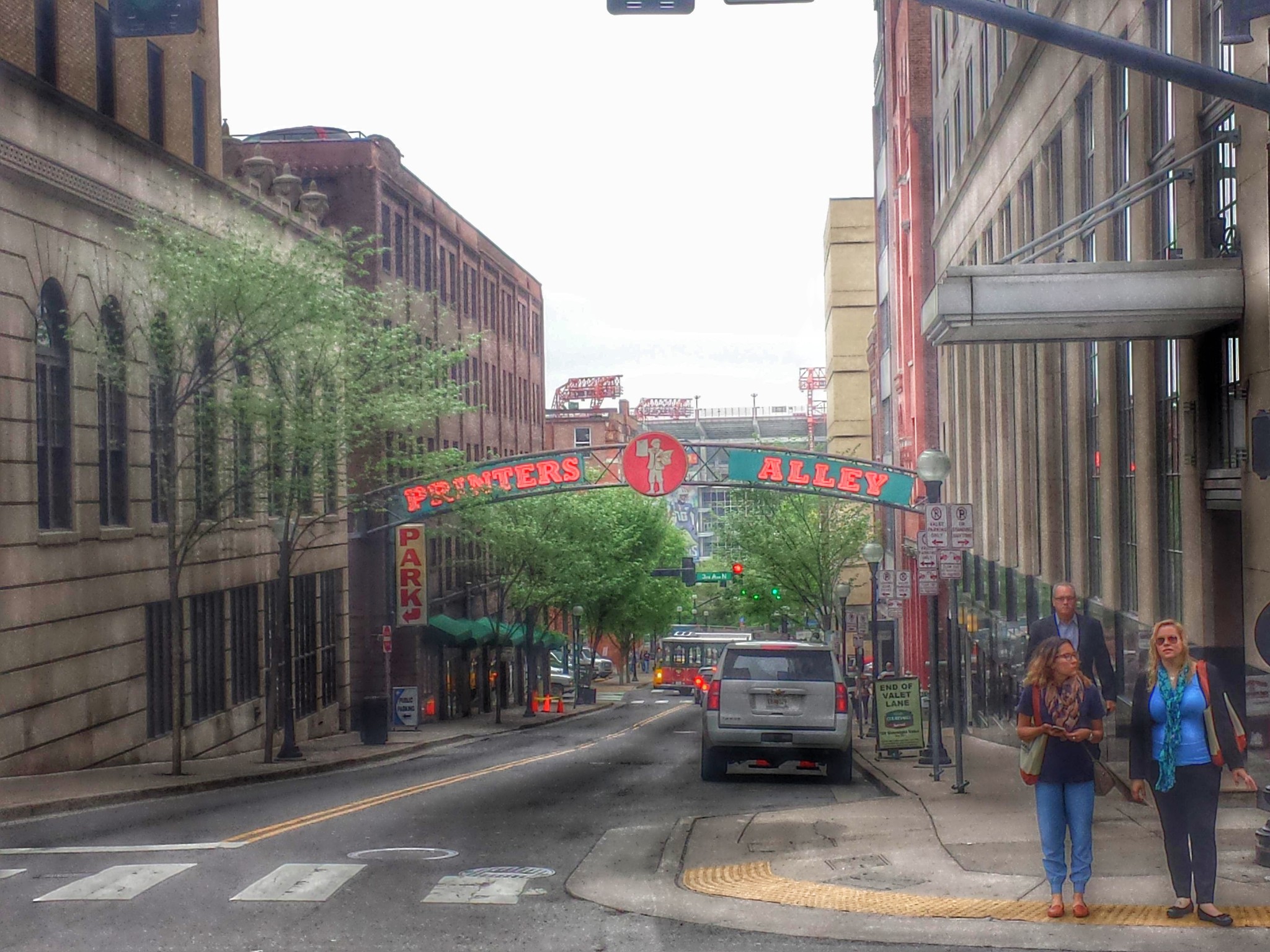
[1197,905,1233,926]
[1048,902,1064,917]
[1072,902,1088,917]
[1167,901,1194,919]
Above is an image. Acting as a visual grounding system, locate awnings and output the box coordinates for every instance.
[426,612,569,652]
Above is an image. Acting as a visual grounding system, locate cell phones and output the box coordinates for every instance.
[1105,708,1110,717]
[1052,725,1066,733]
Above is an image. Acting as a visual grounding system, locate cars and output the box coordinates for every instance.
[692,665,715,704]
[700,672,715,704]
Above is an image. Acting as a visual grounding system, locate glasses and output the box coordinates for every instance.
[1155,636,1180,645]
[1054,651,1078,659]
[1053,596,1076,602]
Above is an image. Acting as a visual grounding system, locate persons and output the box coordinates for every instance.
[1129,619,1258,930]
[1023,582,1117,765]
[1013,634,1107,918]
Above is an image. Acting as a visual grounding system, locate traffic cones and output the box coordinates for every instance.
[529,690,568,714]
[425,696,435,714]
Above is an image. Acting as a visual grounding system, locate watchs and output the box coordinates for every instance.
[1087,730,1093,742]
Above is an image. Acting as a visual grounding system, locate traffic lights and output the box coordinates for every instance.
[571,603,582,709]
[733,564,742,572]
[772,588,778,594]
[776,595,781,599]
[741,589,747,595]
[754,594,759,600]
[677,593,709,626]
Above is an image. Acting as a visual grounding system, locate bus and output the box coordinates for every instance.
[652,635,751,694]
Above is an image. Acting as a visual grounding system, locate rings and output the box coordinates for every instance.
[1073,736,1075,740]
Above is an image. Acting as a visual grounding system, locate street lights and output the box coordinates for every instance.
[861,542,889,737]
[916,446,953,765]
[838,581,852,682]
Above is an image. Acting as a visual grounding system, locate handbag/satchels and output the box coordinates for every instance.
[1017,684,1049,786]
[1195,659,1247,766]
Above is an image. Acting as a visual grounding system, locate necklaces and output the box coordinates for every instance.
[1167,670,1179,681]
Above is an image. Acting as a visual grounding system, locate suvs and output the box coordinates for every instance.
[525,646,614,696]
[699,641,853,784]
